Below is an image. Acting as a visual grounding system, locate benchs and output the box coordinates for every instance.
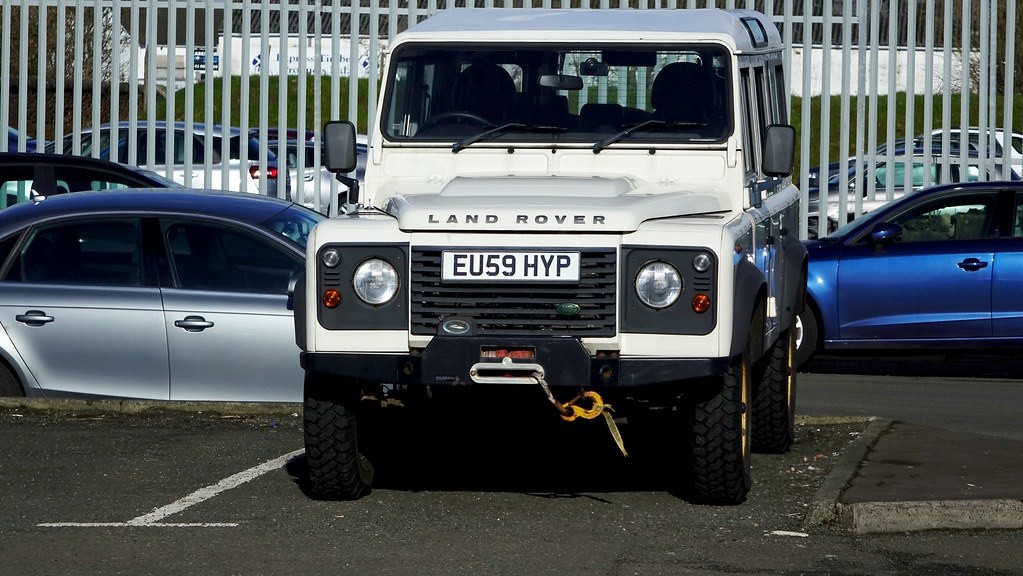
[517,91,652,129]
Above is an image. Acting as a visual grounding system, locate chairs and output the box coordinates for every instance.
[429,63,537,132]
[187,227,250,290]
[633,63,726,135]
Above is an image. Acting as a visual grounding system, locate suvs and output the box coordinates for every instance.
[292,10,809,506]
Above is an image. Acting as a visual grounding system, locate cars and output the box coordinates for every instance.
[792,181,1023,372]
[794,126,1023,239]
[0,120,365,402]
